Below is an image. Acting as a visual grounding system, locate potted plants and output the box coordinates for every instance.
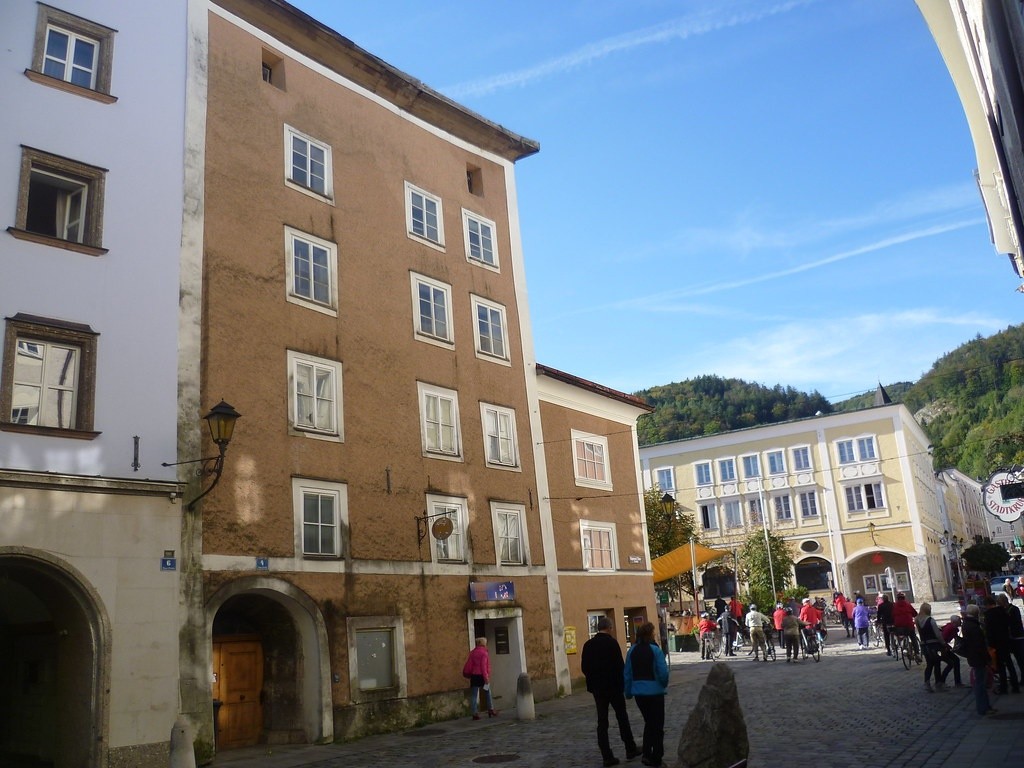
[689,625,700,643]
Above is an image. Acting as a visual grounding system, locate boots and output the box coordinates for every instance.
[924,680,933,692]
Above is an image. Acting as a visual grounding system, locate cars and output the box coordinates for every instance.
[988,575,1020,597]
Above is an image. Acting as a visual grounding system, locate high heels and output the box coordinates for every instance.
[489,709,499,718]
[472,713,481,720]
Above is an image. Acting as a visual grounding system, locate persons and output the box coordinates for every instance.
[714,593,729,631]
[726,594,743,629]
[745,604,770,661]
[1002,576,1024,605]
[716,605,740,656]
[891,592,951,693]
[833,588,895,655]
[658,616,668,665]
[624,621,669,768]
[952,573,991,606]
[582,618,642,766]
[697,613,716,660]
[773,595,827,663]
[938,594,1024,716]
[469,637,499,720]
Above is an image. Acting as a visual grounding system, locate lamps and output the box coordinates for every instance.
[161,397,241,512]
[941,530,964,547]
[867,521,879,546]
[649,492,675,542]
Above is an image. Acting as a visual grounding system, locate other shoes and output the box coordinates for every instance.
[982,708,998,714]
[916,656,922,662]
[886,651,891,656]
[954,682,966,689]
[602,757,619,768]
[700,650,800,663]
[642,753,667,768]
[624,745,643,761]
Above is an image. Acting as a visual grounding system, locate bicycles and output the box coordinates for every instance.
[899,616,924,671]
[814,621,824,654]
[705,631,723,662]
[825,604,900,661]
[797,623,821,663]
[760,622,777,661]
[720,627,755,656]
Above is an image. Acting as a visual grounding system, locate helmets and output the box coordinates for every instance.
[785,607,793,611]
[700,612,709,617]
[802,598,810,603]
[857,599,863,603]
[750,604,757,609]
[897,592,906,599]
[725,605,731,609]
[777,603,783,609]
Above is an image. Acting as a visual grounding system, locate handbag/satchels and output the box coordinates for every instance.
[970,666,993,690]
[1006,653,1022,683]
[463,659,473,679]
[927,646,947,662]
[986,647,998,674]
[954,638,967,658]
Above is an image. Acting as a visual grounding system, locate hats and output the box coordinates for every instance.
[882,595,888,600]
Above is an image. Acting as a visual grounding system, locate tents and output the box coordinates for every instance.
[651,536,737,619]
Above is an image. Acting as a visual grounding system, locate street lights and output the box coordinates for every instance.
[943,529,968,606]
